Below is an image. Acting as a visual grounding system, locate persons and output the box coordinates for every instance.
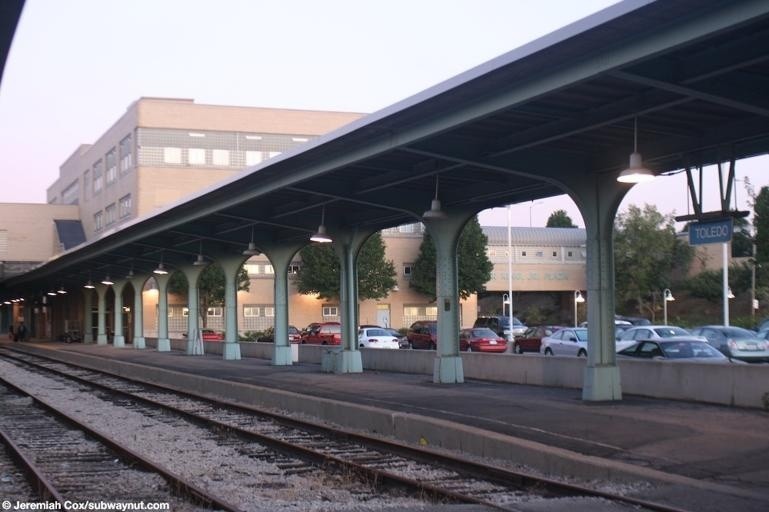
[17,321,28,342]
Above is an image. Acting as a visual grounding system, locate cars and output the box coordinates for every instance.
[357,315,769,364]
[288,325,304,344]
[183,329,224,340]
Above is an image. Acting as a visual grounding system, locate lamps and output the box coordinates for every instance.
[307,204,333,245]
[422,172,449,222]
[152,249,171,275]
[617,114,657,184]
[240,218,261,258]
[191,235,211,268]
[0,266,115,306]
[125,258,135,280]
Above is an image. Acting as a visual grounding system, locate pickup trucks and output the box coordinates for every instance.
[301,321,342,345]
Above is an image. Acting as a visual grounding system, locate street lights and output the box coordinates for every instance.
[503,293,510,317]
[574,290,585,327]
[663,288,676,325]
